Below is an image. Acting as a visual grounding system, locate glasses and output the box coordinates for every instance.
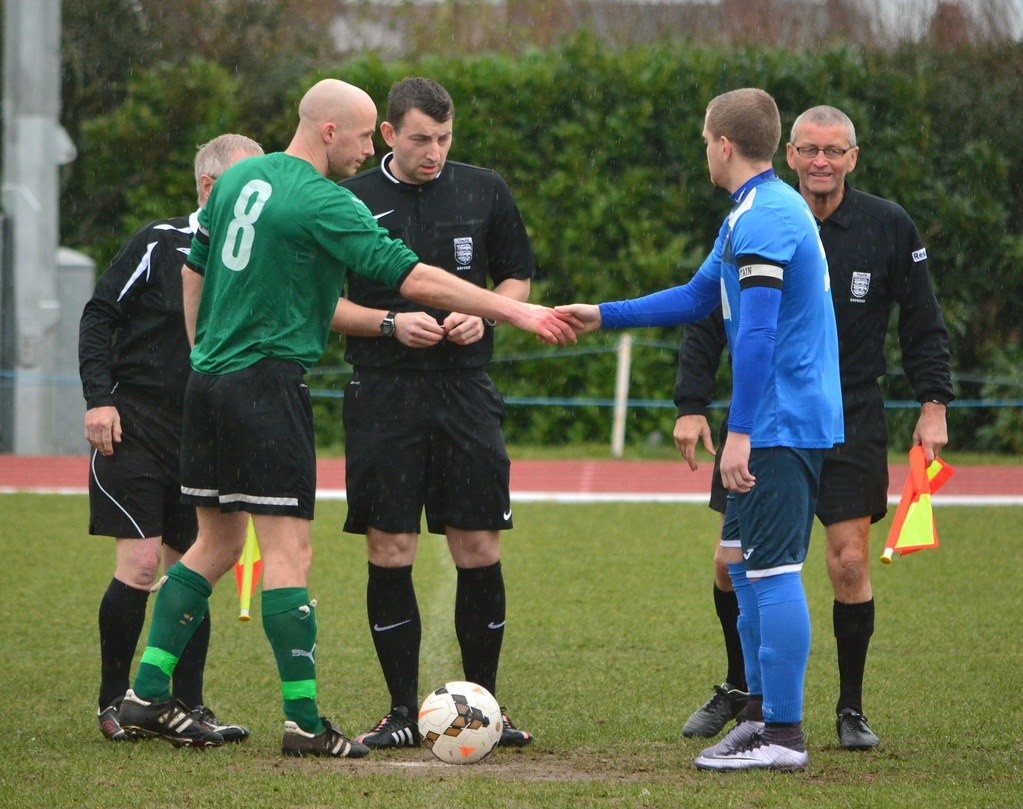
[791,142,853,159]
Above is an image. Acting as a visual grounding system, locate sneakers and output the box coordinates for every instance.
[97,705,124,740]
[683,683,750,738]
[172,698,250,744]
[119,689,224,749]
[700,719,765,756]
[356,705,421,749]
[693,729,808,771]
[836,711,880,748]
[281,716,370,758]
[499,706,533,747]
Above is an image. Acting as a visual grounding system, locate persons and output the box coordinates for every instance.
[536,87,846,771]
[333,77,534,747]
[119,77,587,759]
[77,133,265,741]
[671,104,954,749]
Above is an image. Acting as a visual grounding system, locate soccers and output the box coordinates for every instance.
[418,680,504,765]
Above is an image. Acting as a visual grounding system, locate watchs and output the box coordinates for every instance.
[380,311,398,338]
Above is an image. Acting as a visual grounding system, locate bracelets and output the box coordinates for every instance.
[482,317,497,328]
[933,400,942,404]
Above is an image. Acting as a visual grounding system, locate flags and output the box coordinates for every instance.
[232,512,263,599]
[882,445,955,557]
